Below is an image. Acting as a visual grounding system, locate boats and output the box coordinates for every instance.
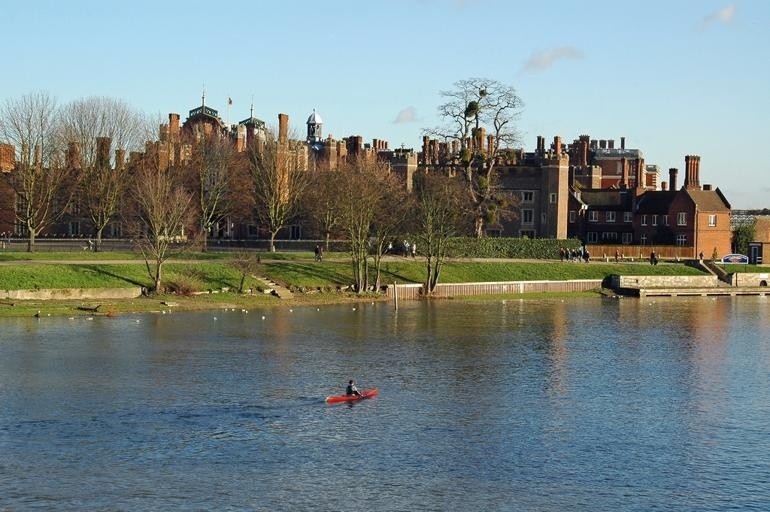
[325,386,377,403]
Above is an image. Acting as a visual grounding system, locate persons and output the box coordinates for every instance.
[559,246,590,263]
[385,237,395,254]
[0,230,12,244]
[314,244,320,261]
[650,250,657,265]
[615,249,619,263]
[699,251,703,259]
[346,379,363,398]
[411,240,416,258]
[402,239,409,257]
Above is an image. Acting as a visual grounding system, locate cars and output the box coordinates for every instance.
[370,239,411,255]
